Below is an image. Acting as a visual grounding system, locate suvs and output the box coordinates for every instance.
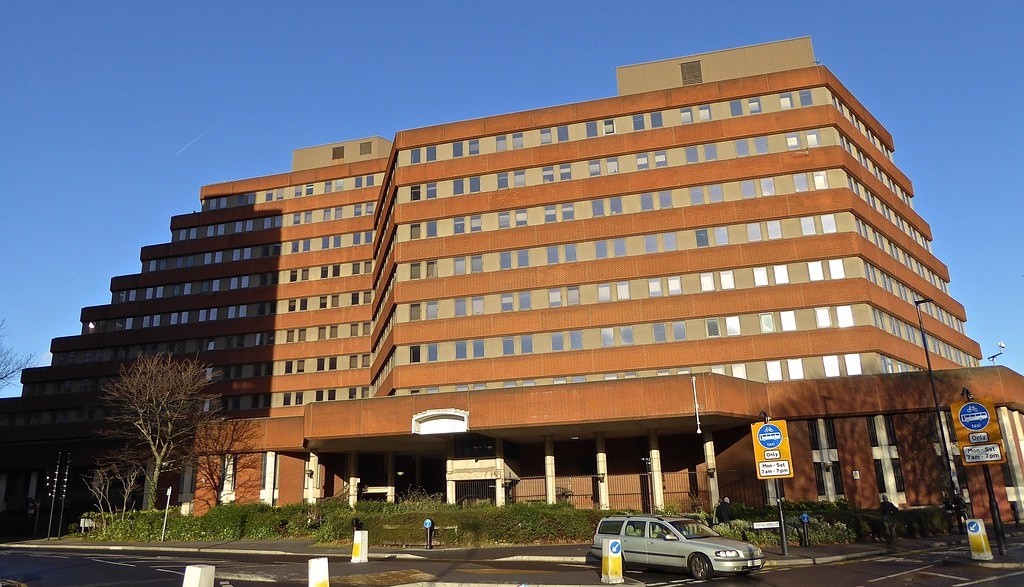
[589,513,767,580]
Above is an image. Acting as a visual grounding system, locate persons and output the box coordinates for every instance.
[880,495,898,544]
[944,491,969,535]
[712,496,737,525]
[26,495,44,534]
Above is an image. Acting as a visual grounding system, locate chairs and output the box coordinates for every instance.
[634,524,644,537]
[626,525,640,537]
[652,524,665,538]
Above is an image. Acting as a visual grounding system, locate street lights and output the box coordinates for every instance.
[913,298,966,535]
